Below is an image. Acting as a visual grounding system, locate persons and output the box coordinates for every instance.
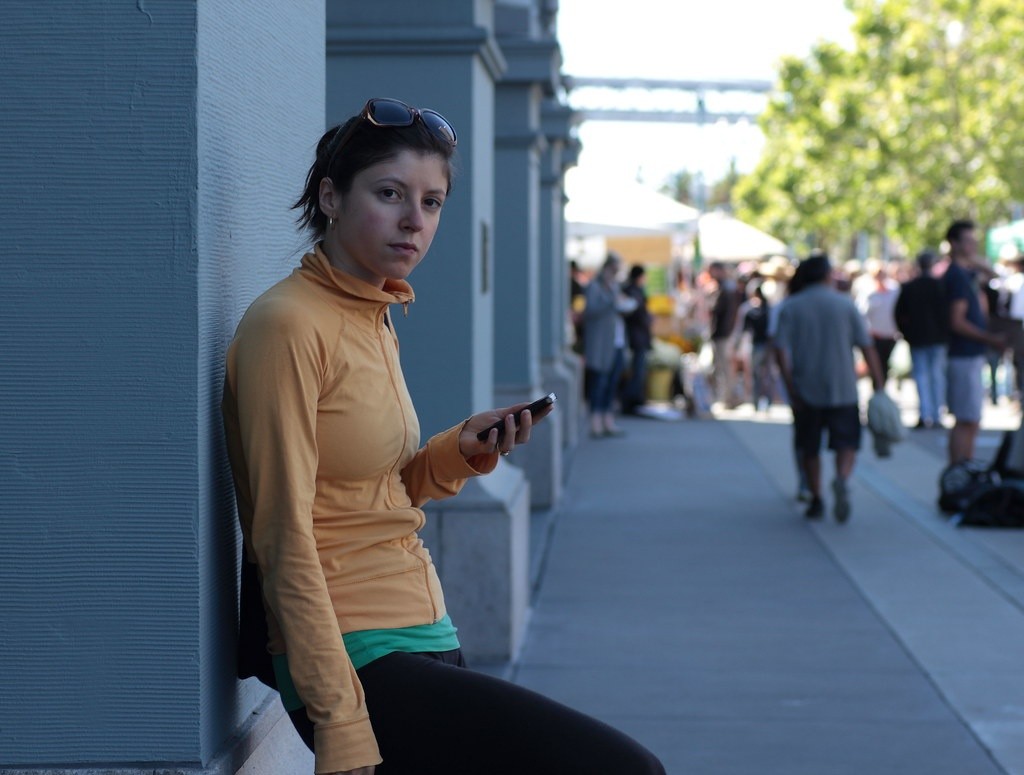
[218,98,665,775]
[568,218,1024,523]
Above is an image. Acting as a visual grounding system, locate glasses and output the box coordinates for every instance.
[326,97,458,178]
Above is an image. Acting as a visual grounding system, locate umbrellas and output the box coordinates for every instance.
[677,207,785,261]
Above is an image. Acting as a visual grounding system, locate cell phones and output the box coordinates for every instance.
[477,393,559,439]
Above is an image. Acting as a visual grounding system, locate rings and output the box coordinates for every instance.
[497,443,509,456]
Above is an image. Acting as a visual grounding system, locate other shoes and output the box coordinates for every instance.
[832,478,849,523]
[805,496,823,518]
